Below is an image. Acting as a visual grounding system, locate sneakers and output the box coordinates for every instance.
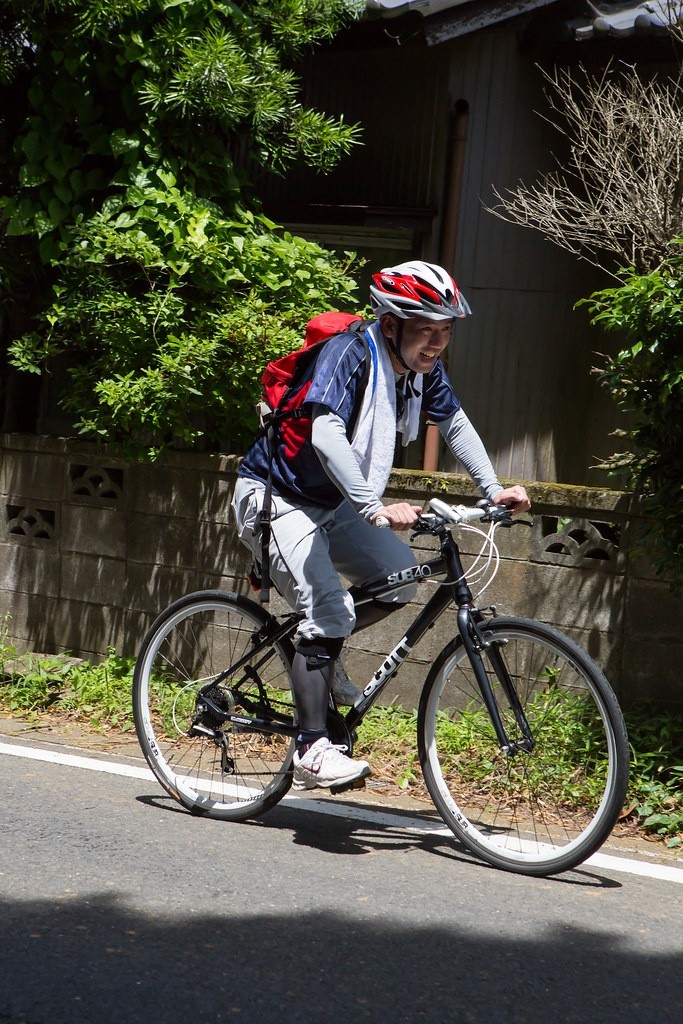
[330,655,362,706]
[291,736,372,790]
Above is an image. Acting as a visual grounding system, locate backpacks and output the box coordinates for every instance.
[262,313,370,464]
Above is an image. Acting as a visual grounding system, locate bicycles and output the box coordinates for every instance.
[131,500,629,876]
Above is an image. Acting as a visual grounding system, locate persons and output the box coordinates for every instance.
[232,262,531,790]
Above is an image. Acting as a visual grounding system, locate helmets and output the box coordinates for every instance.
[370,260,472,320]
